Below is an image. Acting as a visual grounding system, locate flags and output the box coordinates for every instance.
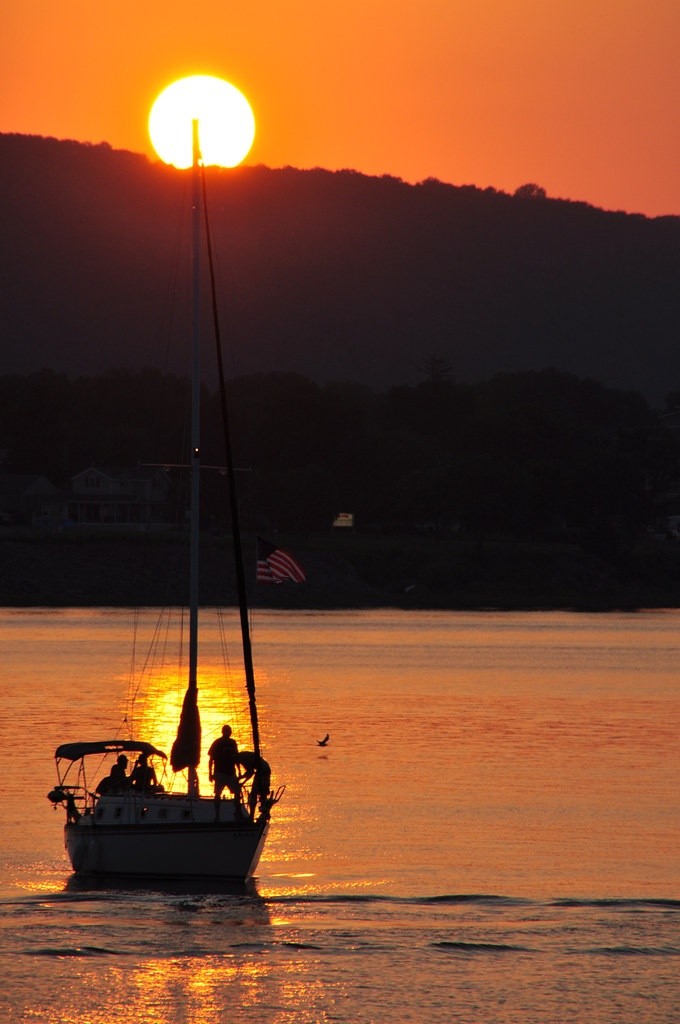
[254,524,306,587]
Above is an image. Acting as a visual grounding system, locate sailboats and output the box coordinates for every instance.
[46,121,287,889]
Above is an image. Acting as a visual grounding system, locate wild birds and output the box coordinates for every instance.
[315,733,331,747]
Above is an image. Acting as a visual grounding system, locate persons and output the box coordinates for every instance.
[236,751,272,822]
[129,754,165,795]
[110,754,134,793]
[208,724,241,802]
